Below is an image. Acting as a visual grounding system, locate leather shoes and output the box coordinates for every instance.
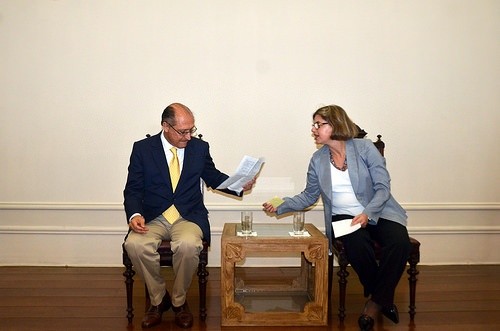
[141,290,192,328]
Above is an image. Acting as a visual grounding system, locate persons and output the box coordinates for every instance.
[262,105,411,331]
[123,103,257,329]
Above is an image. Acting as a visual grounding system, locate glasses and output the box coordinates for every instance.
[312,122,328,130]
[167,122,197,135]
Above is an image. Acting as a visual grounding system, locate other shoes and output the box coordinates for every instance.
[358,299,378,331]
[382,304,400,325]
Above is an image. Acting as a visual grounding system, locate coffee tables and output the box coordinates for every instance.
[221,223,331,327]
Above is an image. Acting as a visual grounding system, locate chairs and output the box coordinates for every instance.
[122,134,209,323]
[328,135,423,320]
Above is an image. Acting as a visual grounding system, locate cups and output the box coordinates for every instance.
[241,212,253,234]
[293,212,305,235]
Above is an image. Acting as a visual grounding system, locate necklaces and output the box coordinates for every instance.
[330,154,347,171]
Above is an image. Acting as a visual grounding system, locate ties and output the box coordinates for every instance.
[162,147,180,225]
